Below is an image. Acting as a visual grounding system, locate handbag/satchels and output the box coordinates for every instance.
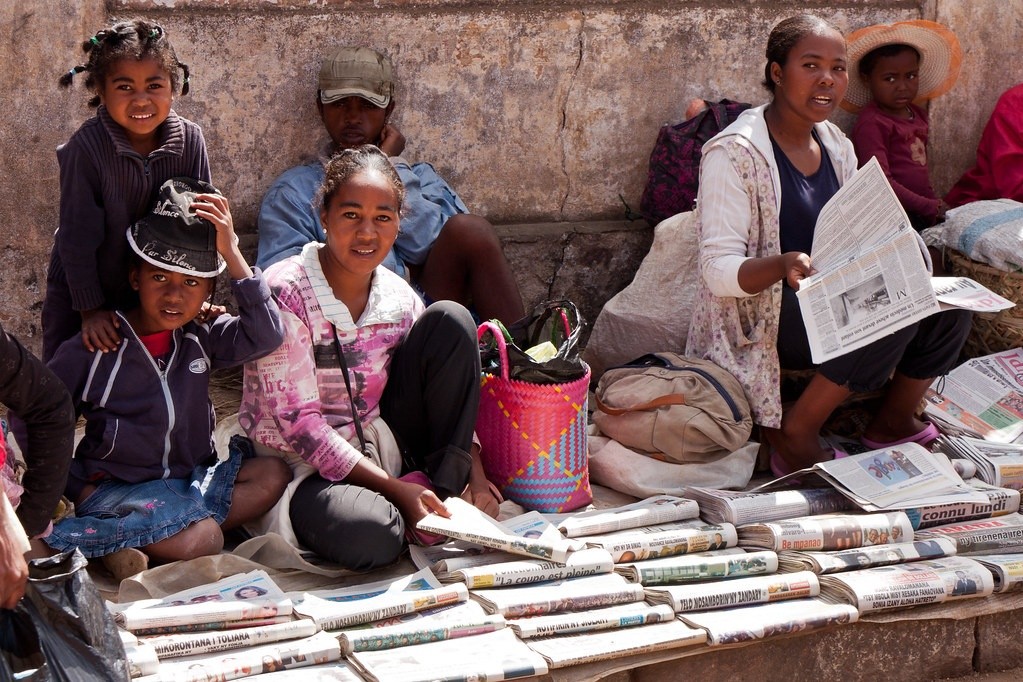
[479,297,594,385]
[0,546,133,682]
[684,136,783,430]
[396,471,446,548]
[594,353,753,464]
[639,99,753,226]
[477,309,594,513]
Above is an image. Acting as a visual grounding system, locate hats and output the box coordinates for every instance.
[126,176,239,279]
[839,19,963,115]
[317,45,395,109]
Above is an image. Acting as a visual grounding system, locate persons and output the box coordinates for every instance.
[260,655,286,673]
[233,585,267,601]
[40,19,213,361]
[830,525,861,549]
[709,533,727,550]
[858,553,870,568]
[952,571,976,596]
[237,144,499,573]
[886,550,900,564]
[0,325,77,610]
[255,47,530,352]
[464,673,485,682]
[6,177,294,582]
[684,13,972,477]
[943,81,1023,210]
[838,19,961,233]
[864,527,903,546]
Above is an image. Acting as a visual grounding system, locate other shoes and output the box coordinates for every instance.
[100,548,149,584]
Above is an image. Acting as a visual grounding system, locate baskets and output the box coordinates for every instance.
[946,245,1023,365]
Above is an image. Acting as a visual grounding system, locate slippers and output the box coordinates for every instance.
[769,444,851,489]
[856,421,943,453]
